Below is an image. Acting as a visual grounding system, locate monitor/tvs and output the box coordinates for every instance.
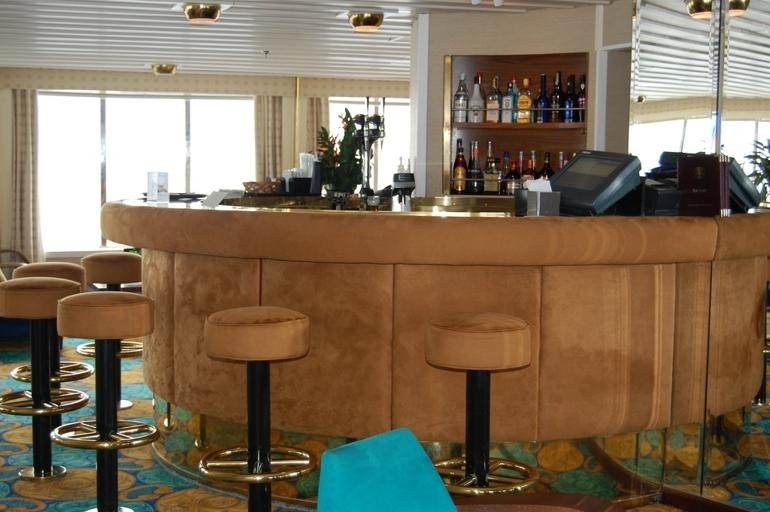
[730,158,763,213]
[550,148,642,217]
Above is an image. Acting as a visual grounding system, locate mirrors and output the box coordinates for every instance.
[628,0,770,215]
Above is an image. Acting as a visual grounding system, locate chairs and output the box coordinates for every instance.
[0,252,64,350]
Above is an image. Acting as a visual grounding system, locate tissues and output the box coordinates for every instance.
[514,178,562,216]
[299,153,314,177]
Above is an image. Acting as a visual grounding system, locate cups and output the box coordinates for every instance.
[367,196,380,212]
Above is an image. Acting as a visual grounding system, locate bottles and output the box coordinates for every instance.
[452,69,587,123]
[452,138,576,195]
[398,157,404,173]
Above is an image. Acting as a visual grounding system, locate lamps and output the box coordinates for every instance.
[169,0,233,23]
[682,0,714,20]
[144,62,180,76]
[728,0,751,17]
[335,8,399,31]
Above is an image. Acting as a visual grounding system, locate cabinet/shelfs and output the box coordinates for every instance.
[443,51,591,198]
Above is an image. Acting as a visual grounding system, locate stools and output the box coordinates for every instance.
[0,278,82,481]
[48,289,160,510]
[9,262,84,432]
[198,306,318,512]
[423,308,540,497]
[80,251,147,411]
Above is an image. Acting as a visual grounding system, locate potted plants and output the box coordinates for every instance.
[309,108,363,195]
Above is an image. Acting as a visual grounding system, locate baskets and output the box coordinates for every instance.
[242,181,282,195]
